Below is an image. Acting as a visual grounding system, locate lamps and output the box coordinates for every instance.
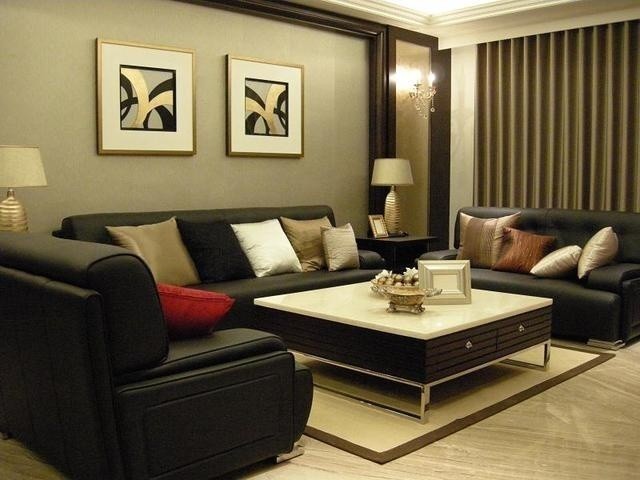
[389,62,439,120]
[370,157,417,239]
[0,143,50,234]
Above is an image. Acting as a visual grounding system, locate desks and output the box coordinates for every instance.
[351,231,444,278]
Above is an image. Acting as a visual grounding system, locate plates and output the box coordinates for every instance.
[370,276,442,299]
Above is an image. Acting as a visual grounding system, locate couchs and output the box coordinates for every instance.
[412,198,639,352]
[0,227,316,478]
[50,202,388,341]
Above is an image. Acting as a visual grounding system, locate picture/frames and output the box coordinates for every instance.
[417,258,473,306]
[95,37,197,157]
[365,212,389,239]
[226,53,305,160]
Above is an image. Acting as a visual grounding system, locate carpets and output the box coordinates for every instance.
[260,334,616,467]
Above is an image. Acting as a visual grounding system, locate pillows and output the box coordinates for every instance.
[528,243,584,278]
[490,224,557,276]
[98,210,364,341]
[575,225,619,282]
[456,207,522,270]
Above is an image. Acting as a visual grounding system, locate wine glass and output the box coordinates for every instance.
[387,286,425,313]
